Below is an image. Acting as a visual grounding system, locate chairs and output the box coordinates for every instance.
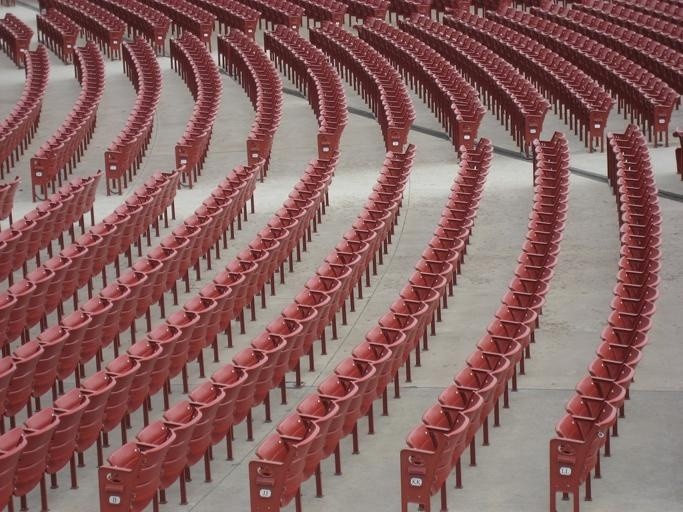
[0,0,681,512]
